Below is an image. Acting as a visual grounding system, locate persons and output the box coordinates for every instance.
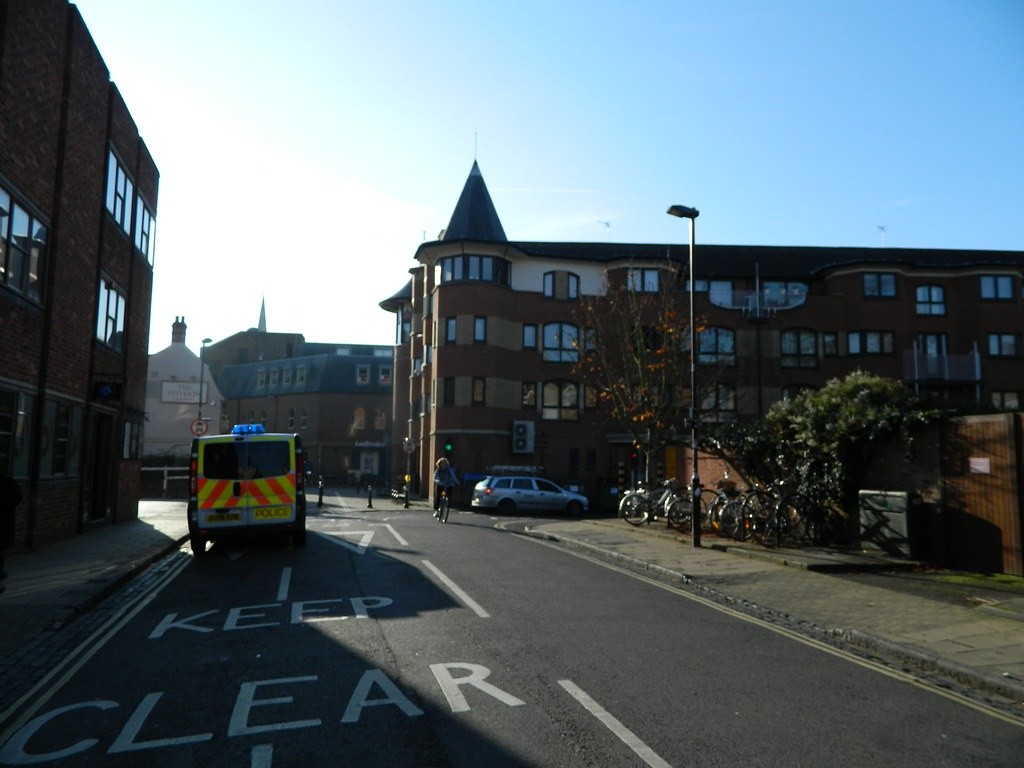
[433,457,461,516]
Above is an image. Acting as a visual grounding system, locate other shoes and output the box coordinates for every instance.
[434,511,439,517]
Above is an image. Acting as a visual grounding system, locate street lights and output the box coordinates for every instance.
[668,204,702,548]
[199,338,212,418]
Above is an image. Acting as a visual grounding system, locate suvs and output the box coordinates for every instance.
[471,475,590,518]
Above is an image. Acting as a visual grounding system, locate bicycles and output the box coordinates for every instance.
[619,477,831,548]
[435,482,458,523]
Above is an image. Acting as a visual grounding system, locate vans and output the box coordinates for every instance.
[188,424,313,556]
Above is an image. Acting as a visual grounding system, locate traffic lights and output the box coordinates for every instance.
[445,438,453,456]
